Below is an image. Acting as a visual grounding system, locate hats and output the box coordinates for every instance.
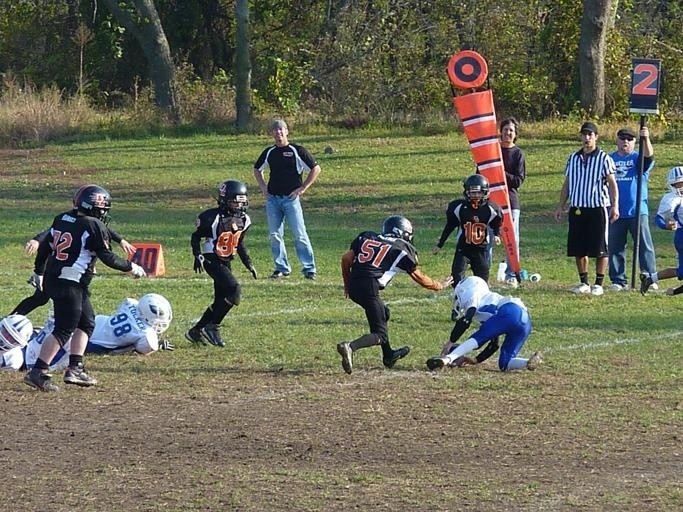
[579,122,597,132]
[272,120,287,129]
[618,128,635,137]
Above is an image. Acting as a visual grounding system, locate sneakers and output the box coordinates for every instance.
[527,351,542,371]
[185,324,226,347]
[639,272,658,295]
[63,366,97,386]
[305,271,316,279]
[572,283,628,295]
[383,346,409,367]
[271,270,290,278]
[337,342,353,374]
[427,357,449,370]
[24,367,58,393]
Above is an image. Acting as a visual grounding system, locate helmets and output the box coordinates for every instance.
[219,181,249,217]
[382,215,413,243]
[454,275,489,316]
[136,292,173,335]
[667,166,683,196]
[73,184,112,226]
[0,314,33,353]
[464,174,490,210]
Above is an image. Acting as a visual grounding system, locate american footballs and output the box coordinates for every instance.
[446,343,465,367]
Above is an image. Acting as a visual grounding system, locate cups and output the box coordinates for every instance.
[668,220,678,230]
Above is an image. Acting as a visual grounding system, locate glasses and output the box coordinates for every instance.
[620,136,633,140]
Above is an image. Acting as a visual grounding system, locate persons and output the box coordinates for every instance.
[337,216,455,374]
[10,185,137,316]
[254,120,322,279]
[22,293,172,372]
[666,285,683,295]
[185,181,257,348]
[24,184,147,394]
[433,174,504,320]
[553,123,620,296]
[476,118,526,284]
[427,276,542,372]
[0,314,33,371]
[640,166,683,295]
[608,126,659,290]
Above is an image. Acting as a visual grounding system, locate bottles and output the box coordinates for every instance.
[497,259,507,282]
[529,273,542,282]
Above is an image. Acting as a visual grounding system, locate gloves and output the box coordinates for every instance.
[129,262,146,279]
[194,254,211,273]
[27,273,43,292]
[159,339,175,351]
[246,262,257,279]
